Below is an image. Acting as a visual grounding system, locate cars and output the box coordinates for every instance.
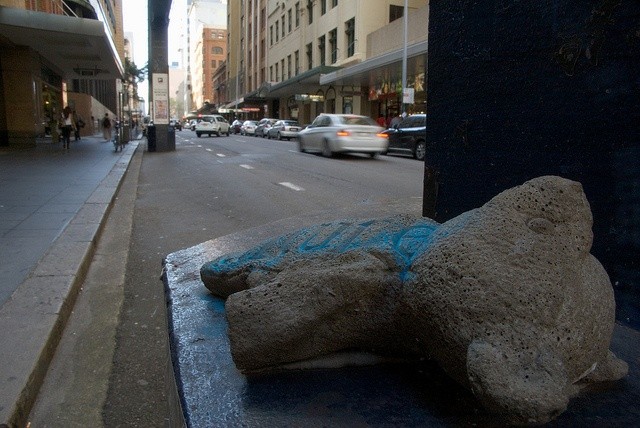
[241,120,259,135]
[297,113,389,159]
[267,120,303,140]
[170,119,175,129]
[230,119,244,134]
[254,118,279,137]
[175,121,182,131]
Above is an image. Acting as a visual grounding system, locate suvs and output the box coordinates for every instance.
[385,114,427,160]
[196,115,230,136]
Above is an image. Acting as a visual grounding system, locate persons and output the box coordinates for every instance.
[72,110,85,141]
[101,113,113,142]
[61,106,78,150]
